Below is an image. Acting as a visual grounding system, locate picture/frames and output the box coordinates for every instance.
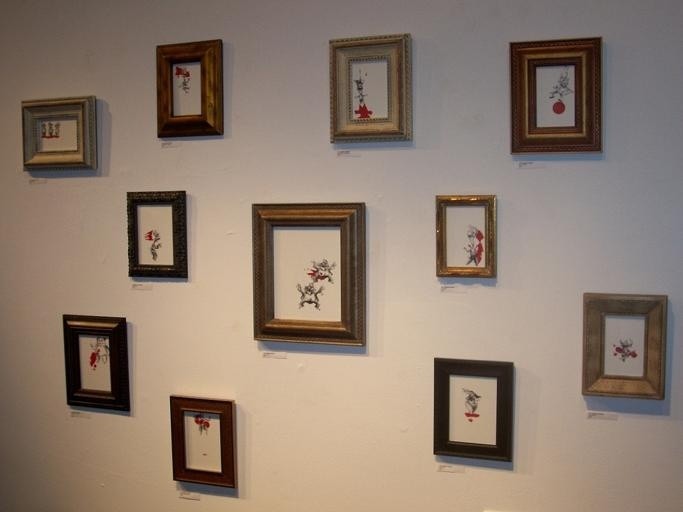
[61,313,132,416]
[250,201,367,347]
[432,356,514,462]
[22,95,101,172]
[434,193,497,280]
[155,38,226,138]
[125,189,190,279]
[507,35,602,154]
[581,291,668,400]
[168,393,239,489]
[327,34,413,145]
